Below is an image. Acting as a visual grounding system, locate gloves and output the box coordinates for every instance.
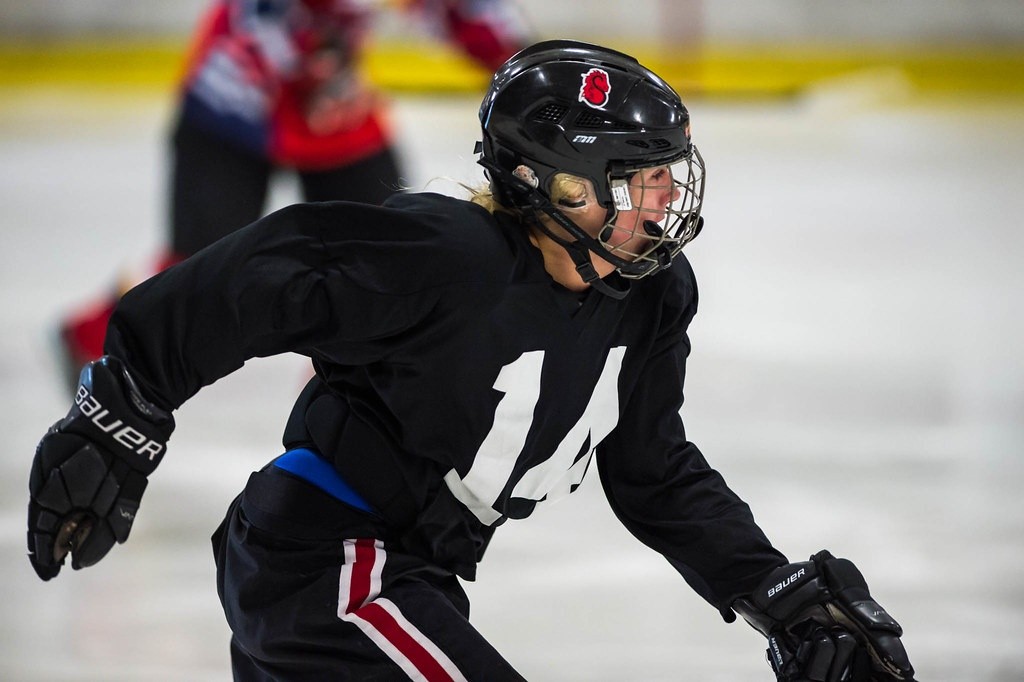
[733,545,914,682]
[29,356,176,581]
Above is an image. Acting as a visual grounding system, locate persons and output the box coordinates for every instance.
[27,41,920,682]
[58,0,544,403]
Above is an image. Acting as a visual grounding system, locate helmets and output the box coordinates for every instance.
[475,40,706,293]
